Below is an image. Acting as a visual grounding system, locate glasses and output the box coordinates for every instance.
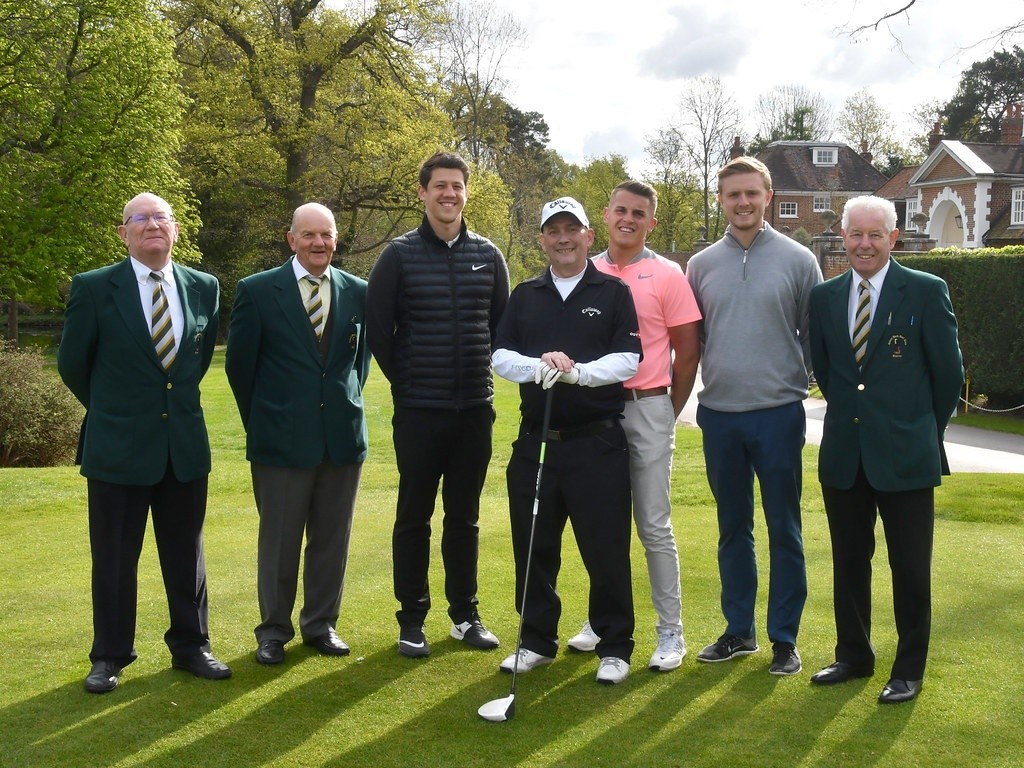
[125,213,173,225]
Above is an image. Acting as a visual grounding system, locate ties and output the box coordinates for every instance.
[303,276,325,342]
[852,278,872,374]
[149,271,177,368]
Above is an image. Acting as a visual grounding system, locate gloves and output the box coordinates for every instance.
[534,362,579,391]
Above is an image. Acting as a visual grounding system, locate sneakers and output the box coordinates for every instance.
[769,642,802,674]
[596,656,630,684]
[498,648,555,673]
[648,632,686,671]
[450,613,500,648]
[695,634,759,662]
[398,625,427,658]
[568,619,601,652]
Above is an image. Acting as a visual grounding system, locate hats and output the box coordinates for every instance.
[539,196,589,235]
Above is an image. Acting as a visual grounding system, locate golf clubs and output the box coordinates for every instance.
[477,383,555,723]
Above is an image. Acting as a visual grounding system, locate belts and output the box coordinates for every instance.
[522,418,615,441]
[624,386,667,401]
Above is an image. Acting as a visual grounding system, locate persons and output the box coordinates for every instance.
[365,151,509,658]
[58,192,231,694]
[686,156,824,675]
[226,202,372,666]
[569,181,703,673]
[809,194,966,703]
[492,195,645,686]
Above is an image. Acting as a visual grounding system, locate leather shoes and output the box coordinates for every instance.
[256,640,284,666]
[877,679,923,704]
[811,662,874,685]
[303,632,350,655]
[85,661,121,692]
[172,651,232,680]
